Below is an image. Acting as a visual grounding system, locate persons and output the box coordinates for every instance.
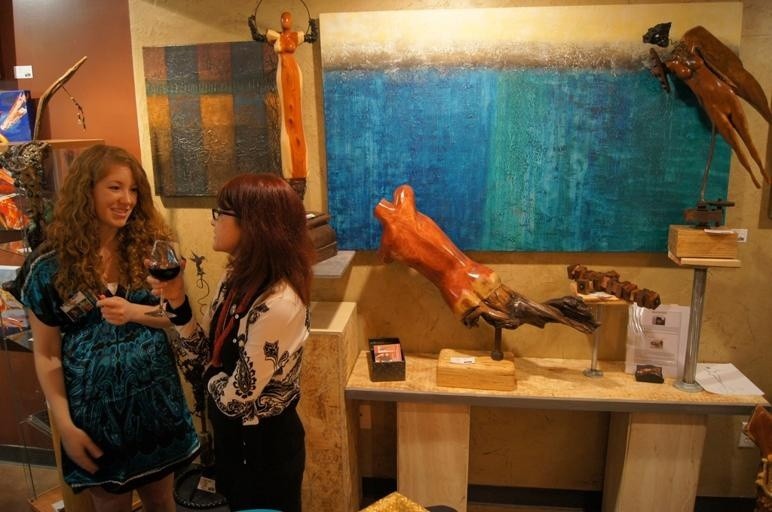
[143,173,310,512]
[21,143,201,512]
[247,12,319,180]
[642,22,763,188]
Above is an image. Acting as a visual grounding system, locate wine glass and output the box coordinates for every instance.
[144,239,181,318]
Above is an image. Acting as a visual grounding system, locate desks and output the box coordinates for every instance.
[344,350,772,512]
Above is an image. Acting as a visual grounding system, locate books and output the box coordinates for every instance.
[301,209,356,280]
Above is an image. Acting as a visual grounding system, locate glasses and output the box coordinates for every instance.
[212,207,238,220]
